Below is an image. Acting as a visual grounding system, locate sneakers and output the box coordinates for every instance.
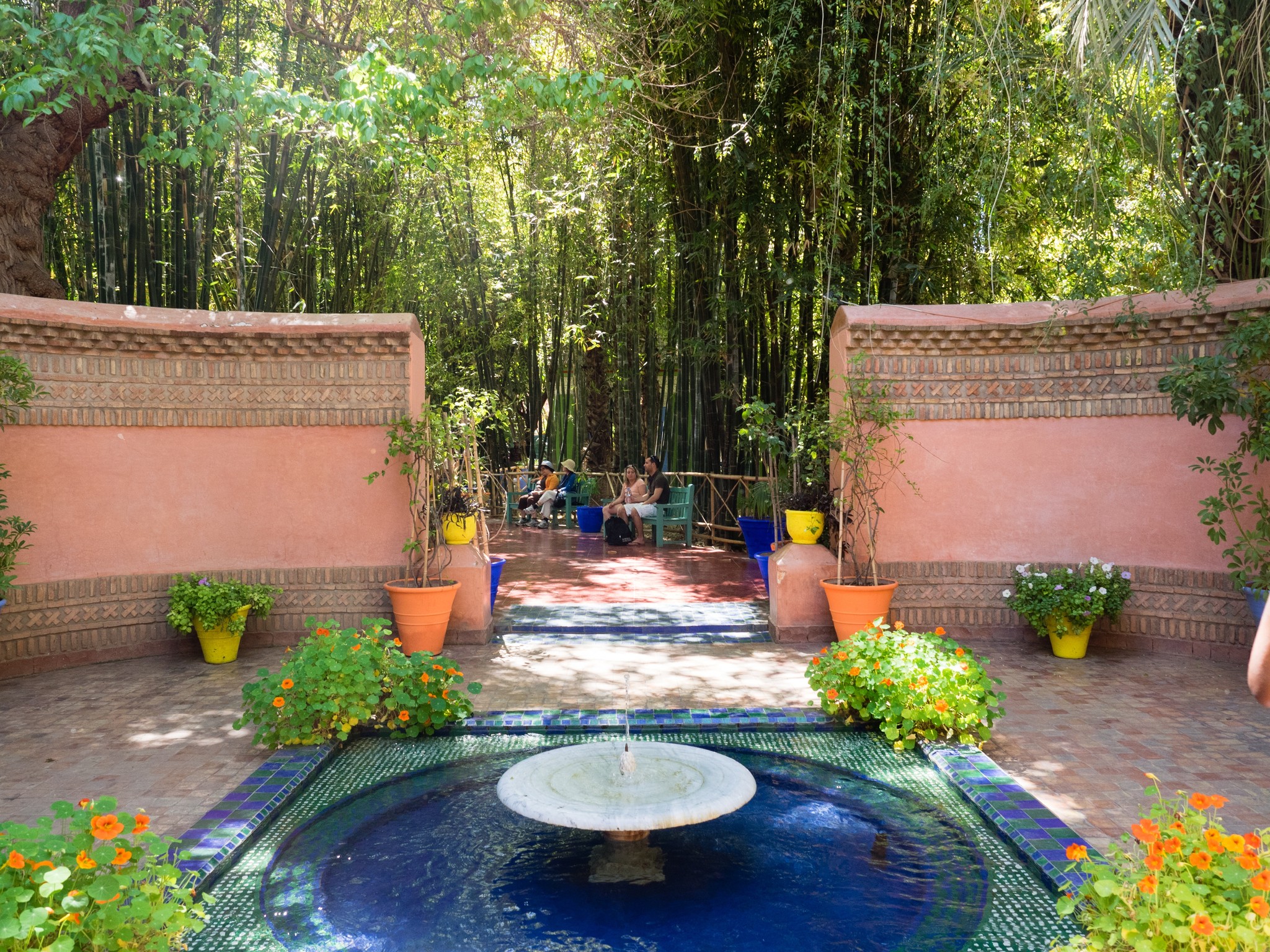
[515,518,527,526]
[523,504,537,514]
[537,519,549,529]
[525,518,539,527]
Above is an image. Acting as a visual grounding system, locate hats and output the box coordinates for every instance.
[561,459,575,472]
[541,460,555,472]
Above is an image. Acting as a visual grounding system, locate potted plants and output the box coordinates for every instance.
[1158,317,1270,622]
[733,352,922,643]
[368,387,505,657]
[165,572,283,663]
[576,477,604,533]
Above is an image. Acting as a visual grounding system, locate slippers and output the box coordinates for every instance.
[602,535,607,541]
[627,541,645,546]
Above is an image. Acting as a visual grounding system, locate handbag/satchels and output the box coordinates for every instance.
[553,491,577,508]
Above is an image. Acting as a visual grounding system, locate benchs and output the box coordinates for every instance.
[507,478,592,529]
[601,484,695,547]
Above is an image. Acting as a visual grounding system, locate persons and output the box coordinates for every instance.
[602,465,649,541]
[1248,582,1269,710]
[503,440,526,477]
[515,460,558,528]
[619,455,670,546]
[523,459,579,529]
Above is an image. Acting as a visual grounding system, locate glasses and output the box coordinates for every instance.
[650,455,657,462]
[540,467,545,471]
[629,464,636,471]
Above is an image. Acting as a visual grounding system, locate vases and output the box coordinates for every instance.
[1045,608,1094,658]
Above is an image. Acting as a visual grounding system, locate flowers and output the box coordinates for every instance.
[1001,555,1135,633]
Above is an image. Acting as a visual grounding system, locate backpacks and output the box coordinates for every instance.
[604,516,634,546]
